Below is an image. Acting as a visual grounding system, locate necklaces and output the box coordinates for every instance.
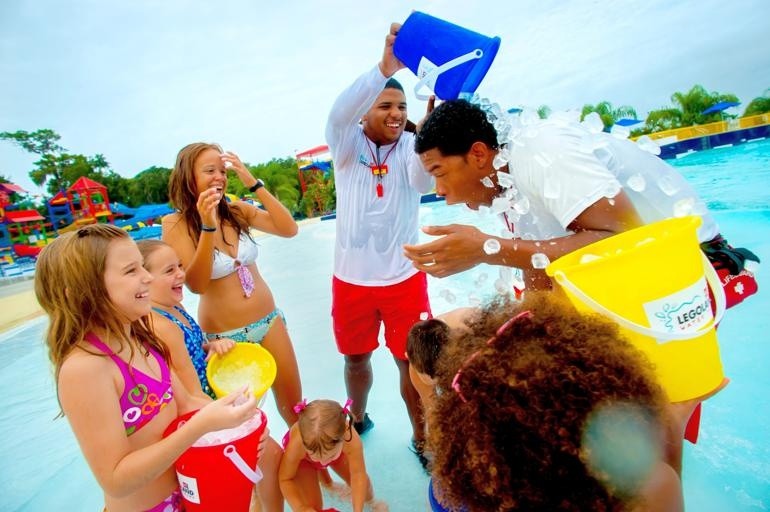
[363,133,398,198]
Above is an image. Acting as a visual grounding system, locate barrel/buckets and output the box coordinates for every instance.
[545,215,723,403]
[161,406,267,512]
[394,10,500,99]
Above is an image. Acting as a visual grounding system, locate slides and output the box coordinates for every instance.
[106,202,177,241]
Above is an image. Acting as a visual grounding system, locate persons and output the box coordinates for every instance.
[402,99,759,443]
[405,304,486,423]
[136,240,286,511]
[422,292,683,509]
[279,400,373,511]
[35,222,271,510]
[324,22,438,470]
[160,142,302,426]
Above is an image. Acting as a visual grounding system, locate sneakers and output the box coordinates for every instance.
[349,413,374,435]
[412,439,433,476]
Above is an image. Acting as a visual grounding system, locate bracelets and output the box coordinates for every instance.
[202,226,216,232]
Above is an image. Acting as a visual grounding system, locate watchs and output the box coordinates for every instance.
[250,179,264,192]
